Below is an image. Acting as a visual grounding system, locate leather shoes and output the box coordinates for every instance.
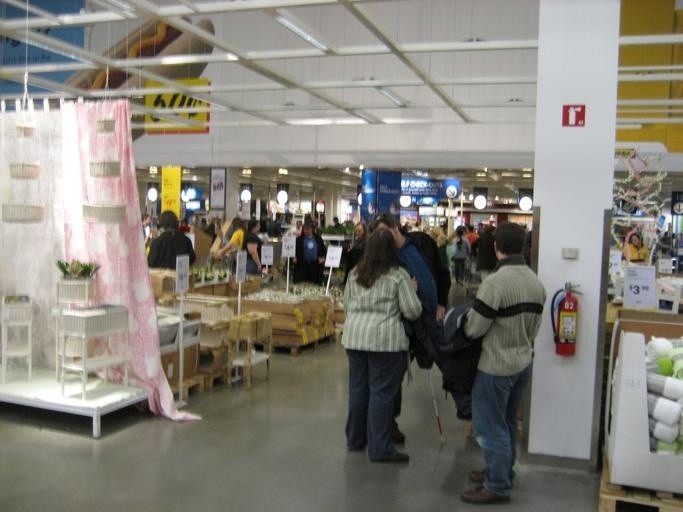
[461,484,510,505]
[470,470,490,481]
[375,450,409,463]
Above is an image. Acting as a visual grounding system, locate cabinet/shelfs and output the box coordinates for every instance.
[224,332,271,390]
[0,294,36,384]
[54,305,136,400]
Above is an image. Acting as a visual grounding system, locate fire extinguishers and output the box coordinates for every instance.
[551,280,583,355]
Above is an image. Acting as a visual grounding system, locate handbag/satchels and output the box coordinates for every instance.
[439,317,483,394]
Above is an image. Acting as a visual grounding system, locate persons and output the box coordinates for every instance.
[373,213,451,369]
[455,221,546,505]
[341,224,420,464]
[655,228,683,251]
[624,228,648,263]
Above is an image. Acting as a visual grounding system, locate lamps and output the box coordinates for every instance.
[146,180,197,203]
[237,181,291,206]
[265,7,413,126]
[470,185,534,213]
[354,185,363,205]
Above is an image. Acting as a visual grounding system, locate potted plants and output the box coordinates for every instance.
[54,258,103,310]
